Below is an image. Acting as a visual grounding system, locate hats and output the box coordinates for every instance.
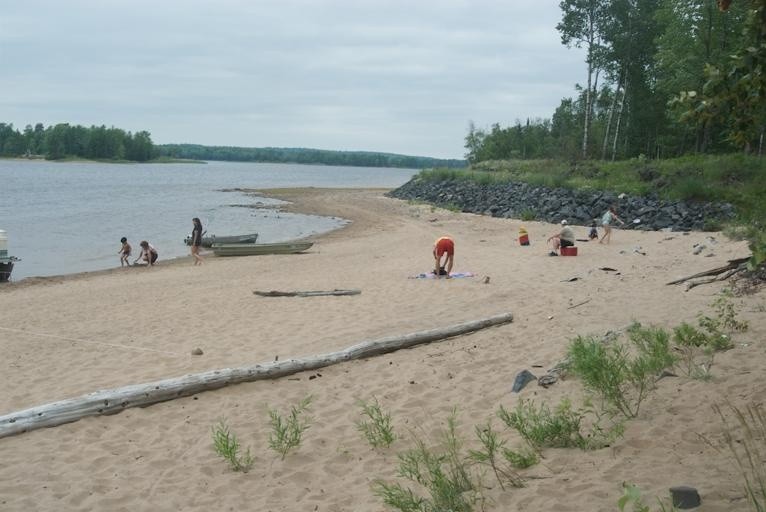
[561,220,568,225]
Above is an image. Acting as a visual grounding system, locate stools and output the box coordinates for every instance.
[560,245,579,255]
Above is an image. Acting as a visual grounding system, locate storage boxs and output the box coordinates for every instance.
[409,270,476,281]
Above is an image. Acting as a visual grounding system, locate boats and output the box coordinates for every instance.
[183,233,259,249]
[209,241,314,256]
[0,229,21,281]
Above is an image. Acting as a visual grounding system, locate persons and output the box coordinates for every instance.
[189,218,203,265]
[133,240,158,267]
[432,236,455,278]
[588,221,596,238]
[117,237,131,267]
[544,218,574,256]
[598,207,621,244]
